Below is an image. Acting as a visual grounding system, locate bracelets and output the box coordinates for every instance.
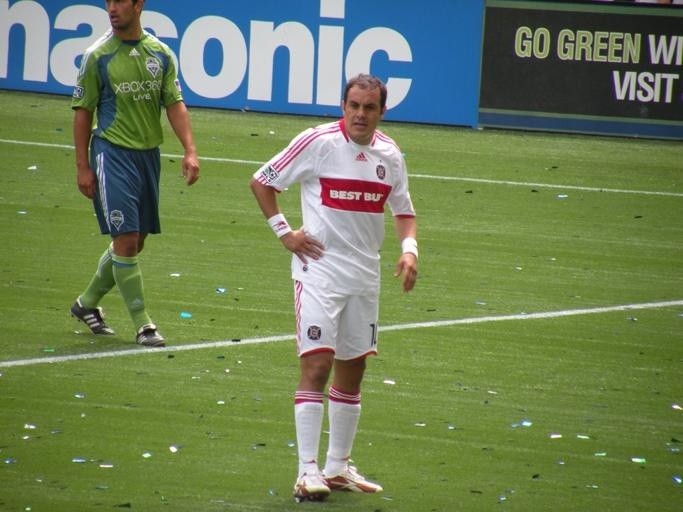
[400,237,417,256]
[264,215,290,239]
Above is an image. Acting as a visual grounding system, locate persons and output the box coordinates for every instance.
[244,73,420,500]
[63,1,199,349]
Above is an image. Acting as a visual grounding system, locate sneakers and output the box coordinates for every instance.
[70,294,115,336]
[321,466,384,494]
[293,471,333,504]
[135,321,166,347]
